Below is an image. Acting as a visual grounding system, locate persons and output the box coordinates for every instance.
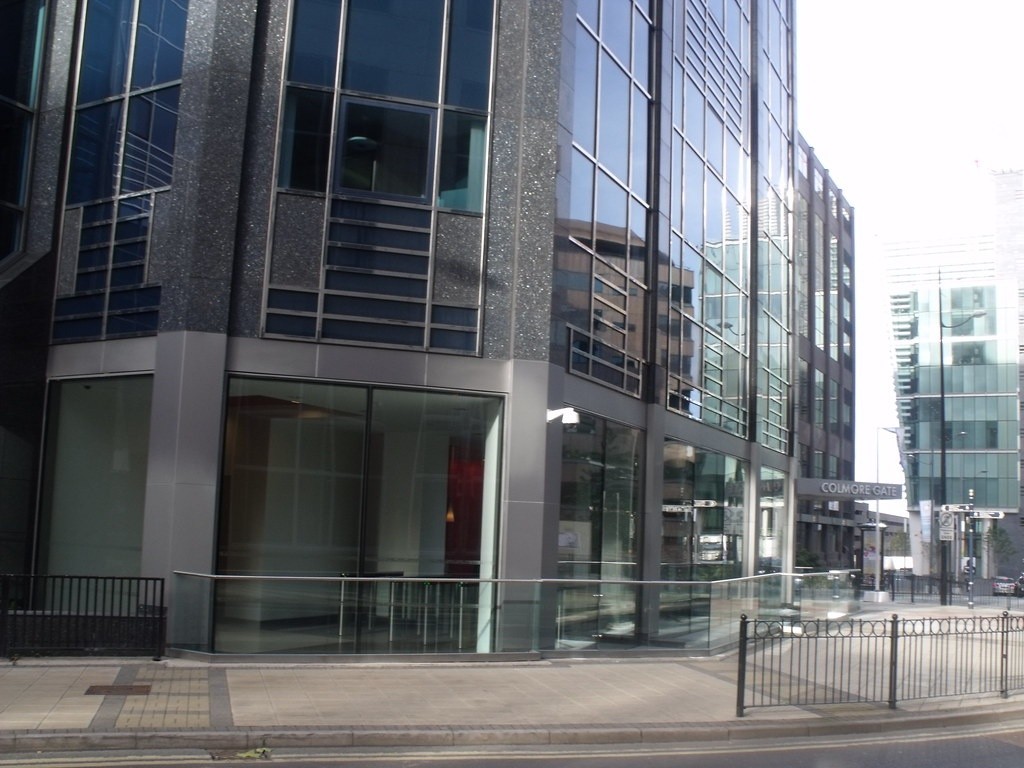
[961,559,976,602]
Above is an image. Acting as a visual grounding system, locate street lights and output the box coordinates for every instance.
[939,272,987,606]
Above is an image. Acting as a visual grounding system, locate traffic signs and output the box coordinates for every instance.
[973,511,1005,519]
[941,504,970,513]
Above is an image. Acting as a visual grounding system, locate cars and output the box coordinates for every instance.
[993,576,1024,597]
